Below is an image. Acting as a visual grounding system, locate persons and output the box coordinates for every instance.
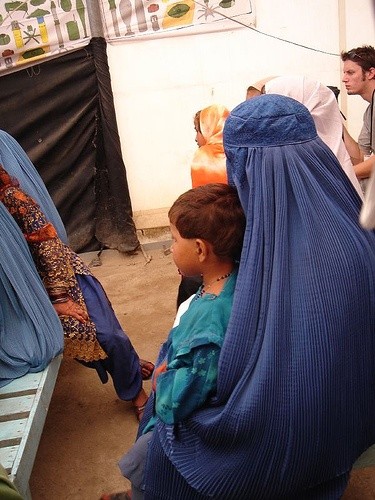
[98,93,374,499]
[176,74,281,308]
[324,45,374,164]
[259,73,365,205]
[134,181,248,439]
[191,104,232,186]
[0,131,155,427]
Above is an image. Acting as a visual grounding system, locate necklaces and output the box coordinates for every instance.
[192,271,230,302]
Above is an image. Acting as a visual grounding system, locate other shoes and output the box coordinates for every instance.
[140,359,155,381]
[131,387,149,422]
[99,489,132,500]
